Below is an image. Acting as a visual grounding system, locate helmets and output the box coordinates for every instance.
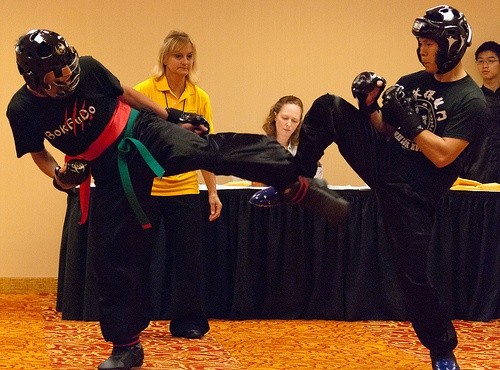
[412,4,472,74]
[15,29,81,99]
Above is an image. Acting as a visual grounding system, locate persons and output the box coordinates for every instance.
[249,5,487,370]
[6,29,349,370]
[460,41,500,184]
[231,95,323,186]
[130,31,222,340]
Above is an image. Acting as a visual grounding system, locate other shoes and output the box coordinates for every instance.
[249,186,282,208]
[283,176,351,227]
[431,351,460,370]
[176,326,204,339]
[97,343,144,370]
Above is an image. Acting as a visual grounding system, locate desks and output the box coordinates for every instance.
[57,183,500,321]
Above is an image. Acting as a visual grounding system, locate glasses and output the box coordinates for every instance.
[475,58,499,64]
